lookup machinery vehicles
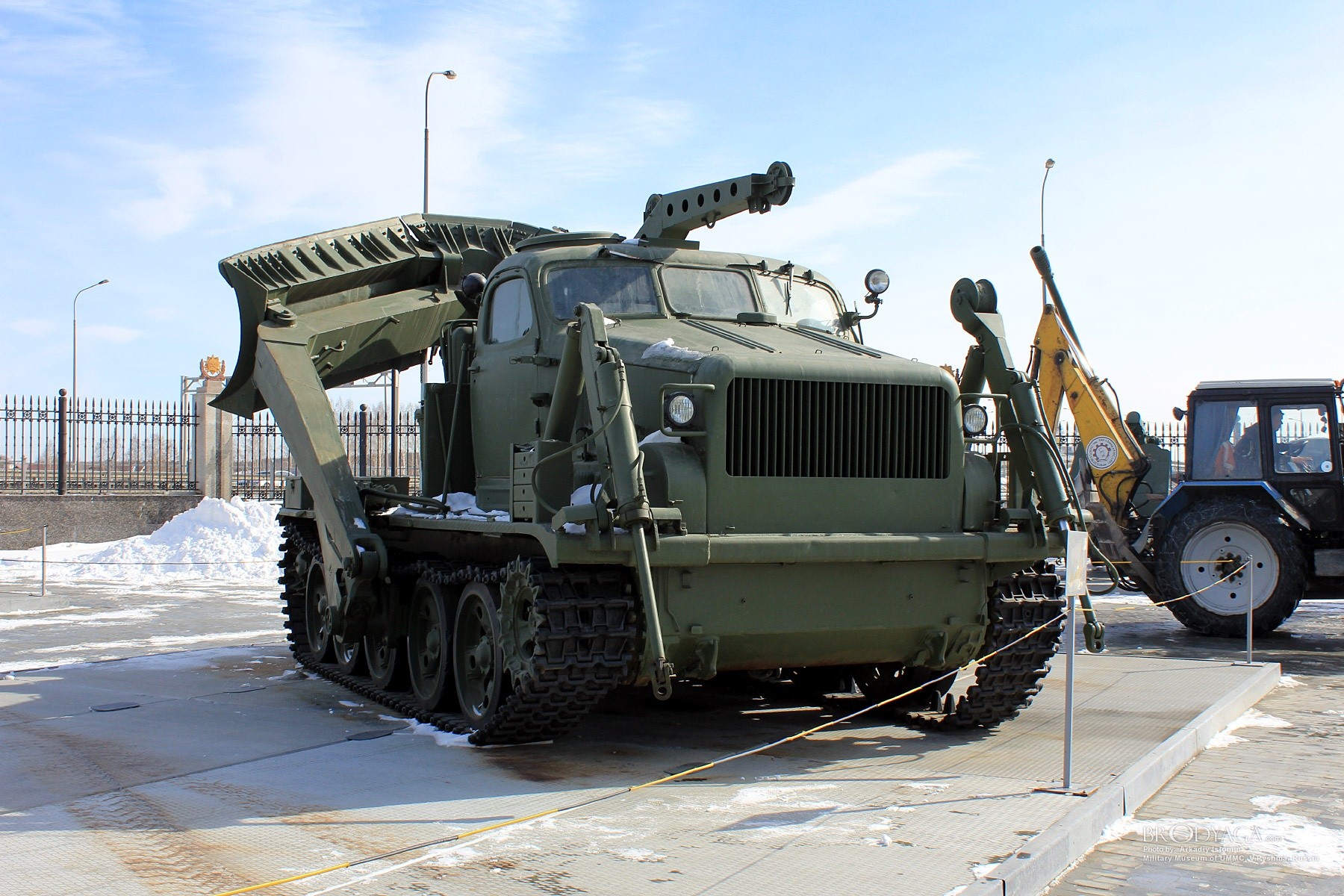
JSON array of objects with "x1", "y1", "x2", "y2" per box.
[
  {"x1": 206, "y1": 159, "x2": 1105, "y2": 749},
  {"x1": 1016, "y1": 301, "x2": 1344, "y2": 639}
]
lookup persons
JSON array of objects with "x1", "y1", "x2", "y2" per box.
[{"x1": 1234, "y1": 408, "x2": 1313, "y2": 478}]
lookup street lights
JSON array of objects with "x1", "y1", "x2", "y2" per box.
[
  {"x1": 424, "y1": 70, "x2": 456, "y2": 213},
  {"x1": 1041, "y1": 158, "x2": 1057, "y2": 306},
  {"x1": 72, "y1": 278, "x2": 109, "y2": 471}
]
